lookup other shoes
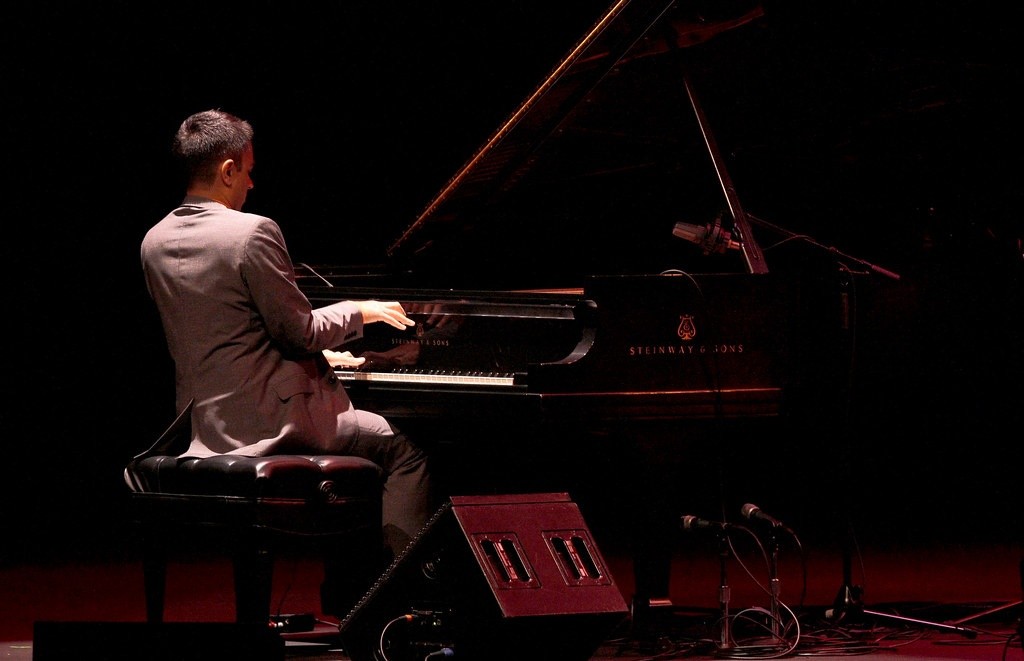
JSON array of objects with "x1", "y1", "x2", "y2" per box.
[
  {"x1": 383, "y1": 558, "x2": 457, "y2": 594},
  {"x1": 320, "y1": 581, "x2": 389, "y2": 615}
]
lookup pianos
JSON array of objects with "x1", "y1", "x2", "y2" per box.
[{"x1": 292, "y1": 1, "x2": 874, "y2": 609}]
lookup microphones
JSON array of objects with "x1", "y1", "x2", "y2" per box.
[
  {"x1": 680, "y1": 515, "x2": 745, "y2": 531},
  {"x1": 673, "y1": 223, "x2": 744, "y2": 251},
  {"x1": 741, "y1": 503, "x2": 793, "y2": 536}
]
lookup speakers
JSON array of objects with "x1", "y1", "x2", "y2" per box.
[{"x1": 337, "y1": 493, "x2": 631, "y2": 661}]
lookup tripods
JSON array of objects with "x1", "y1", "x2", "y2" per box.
[{"x1": 735, "y1": 213, "x2": 977, "y2": 640}]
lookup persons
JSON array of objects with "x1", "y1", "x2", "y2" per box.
[{"x1": 139, "y1": 108, "x2": 454, "y2": 618}]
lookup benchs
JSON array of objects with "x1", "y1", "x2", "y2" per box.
[{"x1": 124, "y1": 455, "x2": 385, "y2": 623}]
